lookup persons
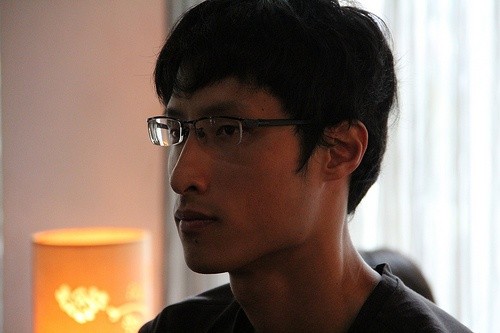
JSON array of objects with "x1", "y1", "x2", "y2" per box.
[{"x1": 137, "y1": 0, "x2": 474, "y2": 333}]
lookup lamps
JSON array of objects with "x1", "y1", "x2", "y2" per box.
[{"x1": 31, "y1": 227, "x2": 155, "y2": 333}]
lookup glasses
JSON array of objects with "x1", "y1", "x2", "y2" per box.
[{"x1": 146, "y1": 113, "x2": 353, "y2": 147}]
